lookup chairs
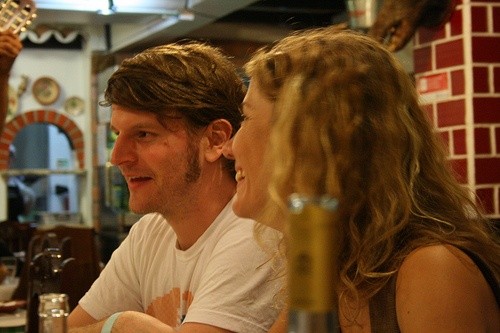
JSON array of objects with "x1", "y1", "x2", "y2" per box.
[
  {"x1": 26, "y1": 225, "x2": 100, "y2": 333},
  {"x1": 0, "y1": 219, "x2": 37, "y2": 257}
]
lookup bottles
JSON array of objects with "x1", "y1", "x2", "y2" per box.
[{"x1": 37, "y1": 293, "x2": 68, "y2": 333}]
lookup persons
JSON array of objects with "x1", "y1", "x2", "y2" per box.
[
  {"x1": 65, "y1": 37, "x2": 284, "y2": 333},
  {"x1": 222, "y1": 24, "x2": 500, "y2": 333}
]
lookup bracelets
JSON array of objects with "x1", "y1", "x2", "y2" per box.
[{"x1": 101, "y1": 312, "x2": 122, "y2": 333}]
